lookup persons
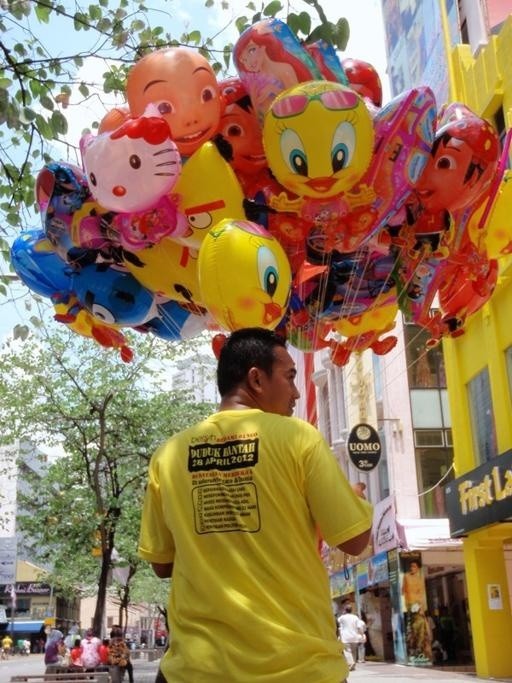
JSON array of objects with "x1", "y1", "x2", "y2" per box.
[
  {"x1": 354, "y1": 611, "x2": 366, "y2": 663},
  {"x1": 137, "y1": 326, "x2": 376, "y2": 681},
  {"x1": 361, "y1": 609, "x2": 376, "y2": 661},
  {"x1": 0, "y1": 622, "x2": 168, "y2": 682},
  {"x1": 400, "y1": 557, "x2": 434, "y2": 654},
  {"x1": 336, "y1": 603, "x2": 366, "y2": 670}
]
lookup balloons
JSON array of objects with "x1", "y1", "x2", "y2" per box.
[{"x1": 11, "y1": 18, "x2": 503, "y2": 365}]
